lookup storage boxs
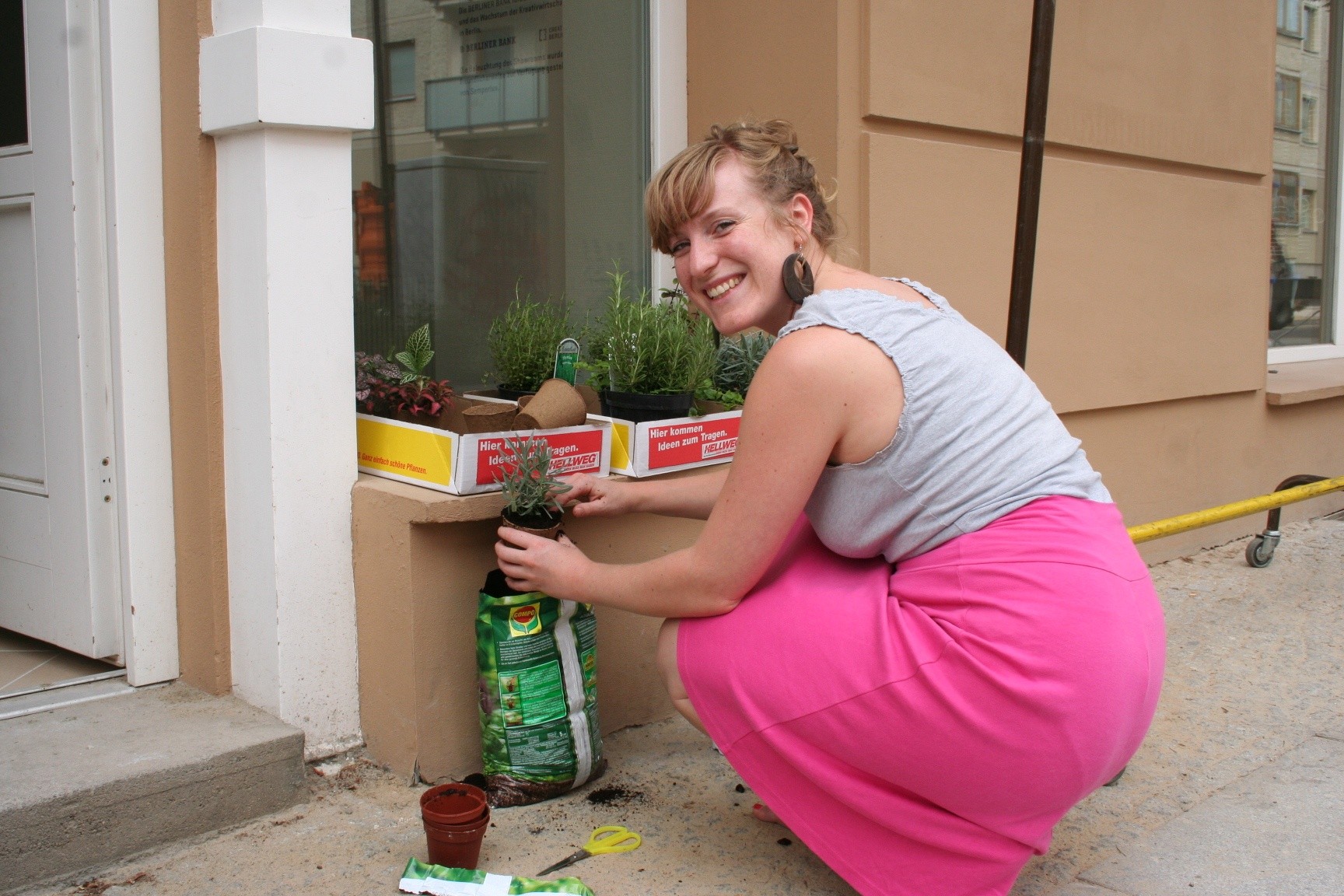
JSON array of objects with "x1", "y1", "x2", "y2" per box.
[
  {"x1": 352, "y1": 391, "x2": 613, "y2": 497},
  {"x1": 462, "y1": 387, "x2": 744, "y2": 477}
]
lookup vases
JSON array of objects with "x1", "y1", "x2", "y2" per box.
[
  {"x1": 419, "y1": 782, "x2": 490, "y2": 870},
  {"x1": 462, "y1": 378, "x2": 588, "y2": 434}
]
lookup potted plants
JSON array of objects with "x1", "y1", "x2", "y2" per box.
[
  {"x1": 492, "y1": 425, "x2": 574, "y2": 562},
  {"x1": 573, "y1": 255, "x2": 718, "y2": 423},
  {"x1": 486, "y1": 275, "x2": 591, "y2": 400},
  {"x1": 378, "y1": 375, "x2": 455, "y2": 428}
]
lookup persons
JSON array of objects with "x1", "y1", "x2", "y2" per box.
[{"x1": 494, "y1": 119, "x2": 1165, "y2": 896}]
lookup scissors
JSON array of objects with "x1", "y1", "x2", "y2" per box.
[{"x1": 536, "y1": 825, "x2": 640, "y2": 877}]
